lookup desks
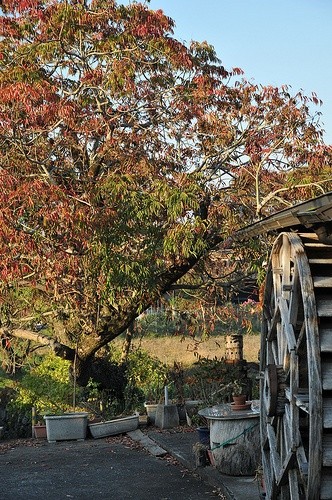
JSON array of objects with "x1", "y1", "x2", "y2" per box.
[{"x1": 198, "y1": 399, "x2": 267, "y2": 476}]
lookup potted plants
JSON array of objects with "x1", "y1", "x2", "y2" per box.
[
  {"x1": 18, "y1": 372, "x2": 180, "y2": 444},
  {"x1": 193, "y1": 416, "x2": 213, "y2": 467},
  {"x1": 230, "y1": 380, "x2": 252, "y2": 410}
]
